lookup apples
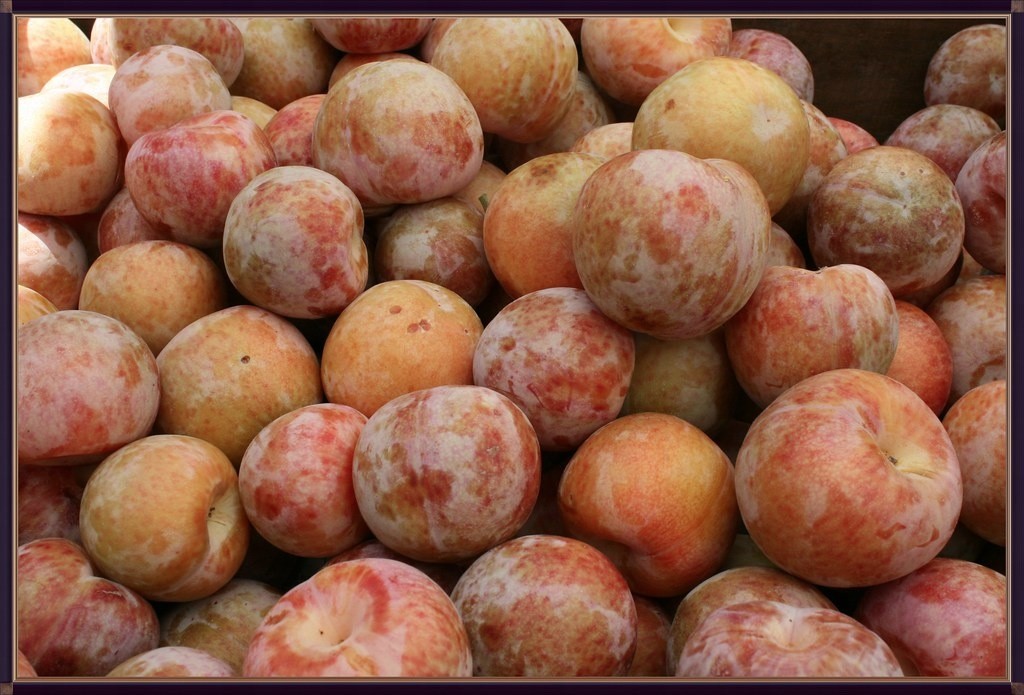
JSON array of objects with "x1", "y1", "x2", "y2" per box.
[{"x1": 16, "y1": 15, "x2": 1010, "y2": 683}]
